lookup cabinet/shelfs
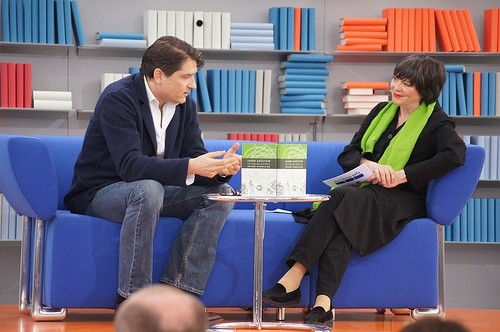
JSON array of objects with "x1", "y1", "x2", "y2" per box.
[
  {"x1": 76, "y1": 45, "x2": 326, "y2": 141},
  {"x1": 0, "y1": 42, "x2": 75, "y2": 243},
  {"x1": 330, "y1": 50, "x2": 500, "y2": 245}
]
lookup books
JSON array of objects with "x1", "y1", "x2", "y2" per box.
[
  {"x1": 1, "y1": 0, "x2": 500, "y2": 143},
  {"x1": 445, "y1": 136, "x2": 500, "y2": 242},
  {"x1": 242, "y1": 143, "x2": 307, "y2": 199}
]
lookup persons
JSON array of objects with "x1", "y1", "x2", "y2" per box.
[
  {"x1": 263, "y1": 53, "x2": 466, "y2": 325},
  {"x1": 63, "y1": 35, "x2": 242, "y2": 310},
  {"x1": 113, "y1": 283, "x2": 208, "y2": 332}
]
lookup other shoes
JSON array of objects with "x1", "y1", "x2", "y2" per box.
[
  {"x1": 262, "y1": 283, "x2": 301, "y2": 307},
  {"x1": 304, "y1": 307, "x2": 333, "y2": 325}
]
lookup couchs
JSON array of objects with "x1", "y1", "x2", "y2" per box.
[{"x1": 0, "y1": 135, "x2": 486, "y2": 320}]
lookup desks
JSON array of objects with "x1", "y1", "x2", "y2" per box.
[{"x1": 200, "y1": 191, "x2": 333, "y2": 332}]
